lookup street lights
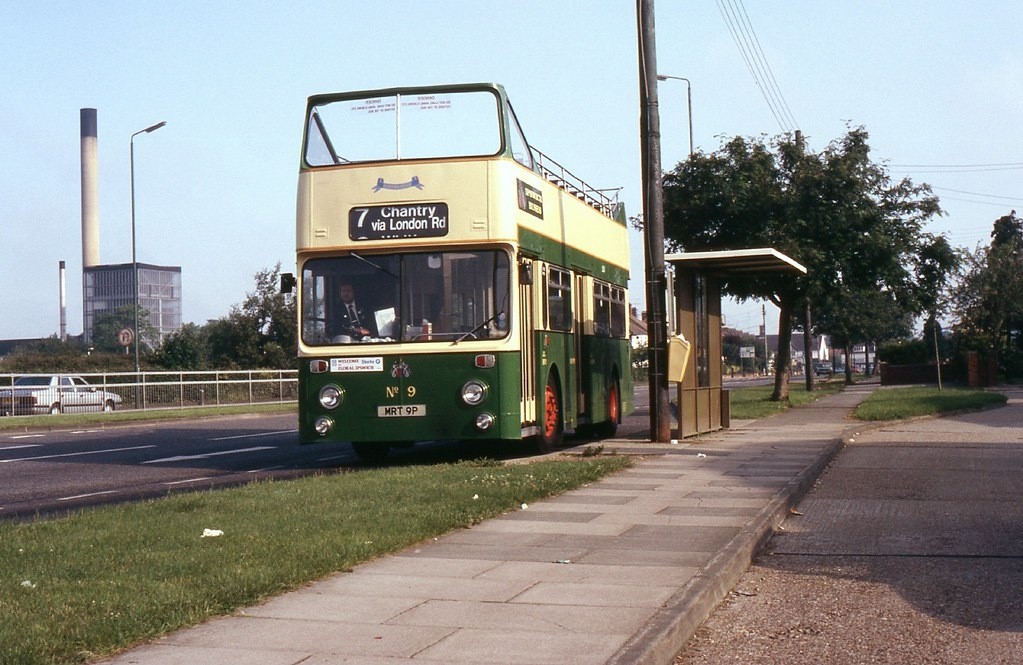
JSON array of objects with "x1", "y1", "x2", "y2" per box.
[
  {"x1": 130, "y1": 121, "x2": 168, "y2": 411},
  {"x1": 658, "y1": 71, "x2": 695, "y2": 171}
]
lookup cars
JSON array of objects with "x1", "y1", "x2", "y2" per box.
[
  {"x1": 817, "y1": 362, "x2": 831, "y2": 376},
  {"x1": 851, "y1": 365, "x2": 863, "y2": 372},
  {"x1": 835, "y1": 368, "x2": 845, "y2": 375},
  {"x1": 0, "y1": 376, "x2": 122, "y2": 416}
]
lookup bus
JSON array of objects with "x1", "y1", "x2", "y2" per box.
[{"x1": 278, "y1": 81, "x2": 635, "y2": 451}]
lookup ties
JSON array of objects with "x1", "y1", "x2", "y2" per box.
[{"x1": 348, "y1": 305, "x2": 361, "y2": 329}]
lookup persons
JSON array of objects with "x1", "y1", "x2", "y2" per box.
[{"x1": 331, "y1": 282, "x2": 378, "y2": 342}]
